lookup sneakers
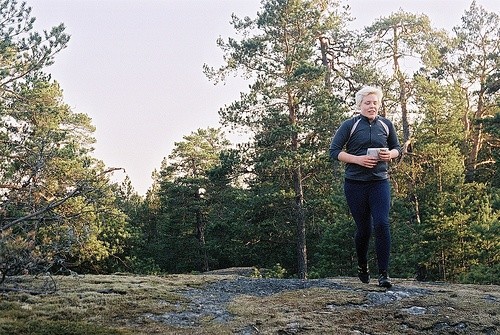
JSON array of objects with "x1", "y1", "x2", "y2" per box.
[
  {"x1": 378, "y1": 270, "x2": 393, "y2": 287},
  {"x1": 357, "y1": 258, "x2": 370, "y2": 284}
]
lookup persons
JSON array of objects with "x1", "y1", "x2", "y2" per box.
[{"x1": 330, "y1": 85, "x2": 401, "y2": 287}]
